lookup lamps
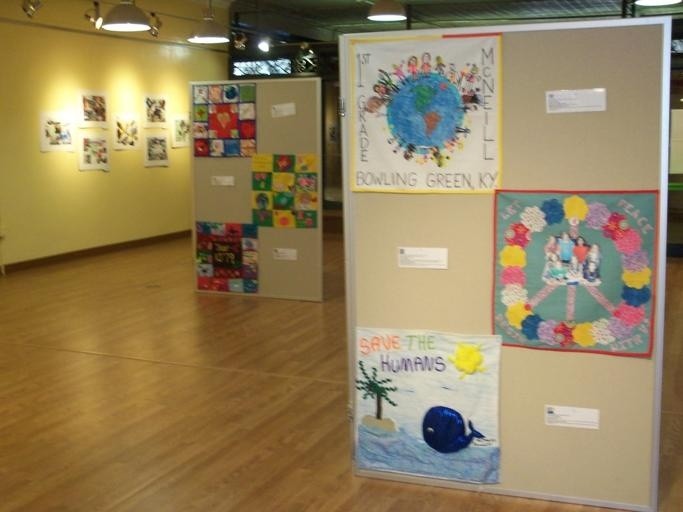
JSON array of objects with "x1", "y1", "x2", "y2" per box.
[
  {"x1": 21, "y1": 1, "x2": 273, "y2": 53},
  {"x1": 365, "y1": 1, "x2": 408, "y2": 22}
]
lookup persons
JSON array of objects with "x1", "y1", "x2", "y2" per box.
[
  {"x1": 537, "y1": 228, "x2": 601, "y2": 289},
  {"x1": 364, "y1": 49, "x2": 481, "y2": 169}
]
